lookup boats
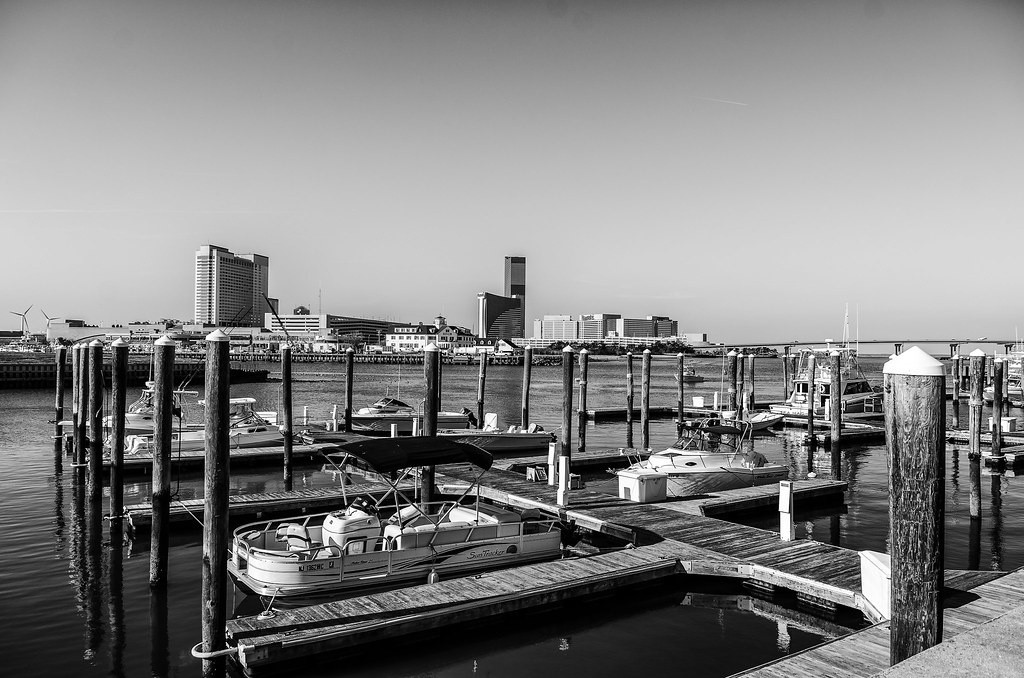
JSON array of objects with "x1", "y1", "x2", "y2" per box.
[
  {"x1": 981, "y1": 326, "x2": 1024, "y2": 405},
  {"x1": 227, "y1": 437, "x2": 567, "y2": 596},
  {"x1": 605, "y1": 414, "x2": 790, "y2": 497},
  {"x1": 53, "y1": 305, "x2": 340, "y2": 469},
  {"x1": 675, "y1": 368, "x2": 709, "y2": 382},
  {"x1": 768, "y1": 305, "x2": 884, "y2": 429},
  {"x1": 676, "y1": 346, "x2": 785, "y2": 439},
  {"x1": 338, "y1": 364, "x2": 557, "y2": 451}
]
